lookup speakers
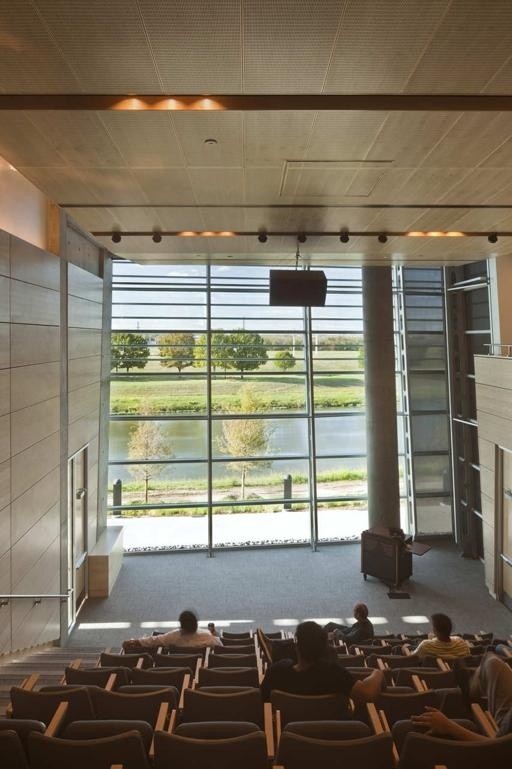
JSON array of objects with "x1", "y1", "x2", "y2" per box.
[{"x1": 270, "y1": 269, "x2": 326, "y2": 306}]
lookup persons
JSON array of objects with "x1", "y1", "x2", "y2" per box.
[
  {"x1": 260, "y1": 622, "x2": 384, "y2": 719},
  {"x1": 322, "y1": 603, "x2": 374, "y2": 646},
  {"x1": 495, "y1": 643, "x2": 512, "y2": 655},
  {"x1": 121, "y1": 612, "x2": 224, "y2": 650},
  {"x1": 411, "y1": 656, "x2": 511, "y2": 743},
  {"x1": 400, "y1": 612, "x2": 472, "y2": 662}
]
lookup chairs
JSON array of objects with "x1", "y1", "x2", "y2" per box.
[{"x1": 1, "y1": 626, "x2": 512, "y2": 768}]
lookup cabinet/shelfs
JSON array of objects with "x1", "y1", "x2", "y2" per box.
[
  {"x1": 88, "y1": 525, "x2": 124, "y2": 598},
  {"x1": 361, "y1": 525, "x2": 432, "y2": 591}
]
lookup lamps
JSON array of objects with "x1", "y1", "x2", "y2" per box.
[{"x1": 90, "y1": 228, "x2": 512, "y2": 244}]
